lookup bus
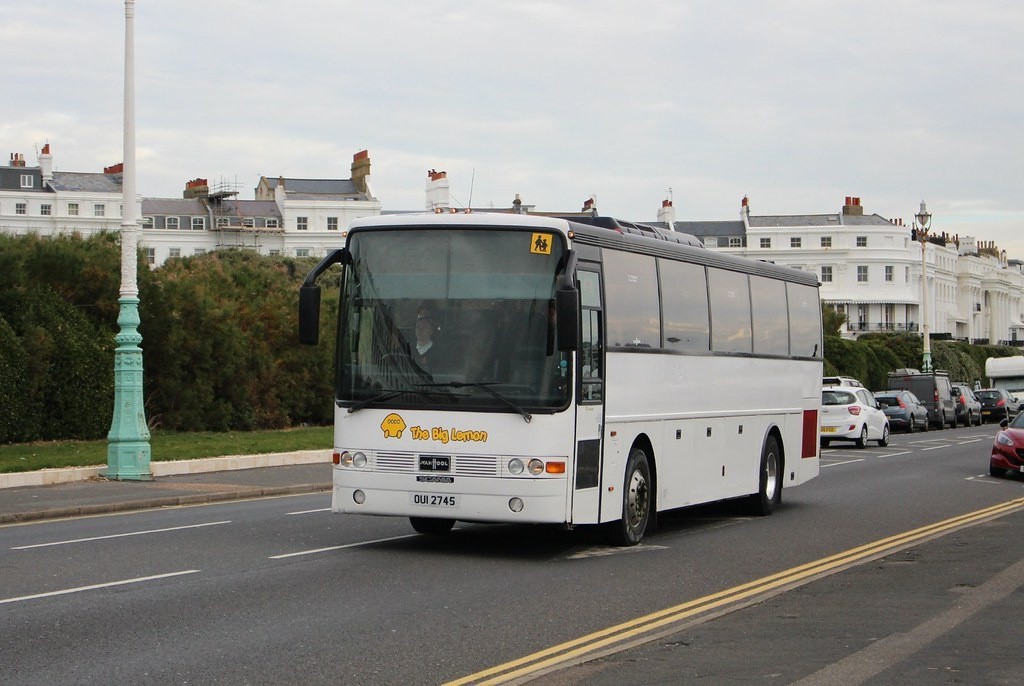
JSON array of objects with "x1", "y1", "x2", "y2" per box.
[{"x1": 300, "y1": 215, "x2": 824, "y2": 543}]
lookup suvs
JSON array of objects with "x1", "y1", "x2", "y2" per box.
[{"x1": 954, "y1": 383, "x2": 981, "y2": 426}]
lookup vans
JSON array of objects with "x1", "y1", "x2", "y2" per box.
[{"x1": 890, "y1": 367, "x2": 956, "y2": 429}]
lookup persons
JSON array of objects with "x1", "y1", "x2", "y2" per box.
[
  {"x1": 414, "y1": 306, "x2": 429, "y2": 323},
  {"x1": 400, "y1": 319, "x2": 440, "y2": 373}
]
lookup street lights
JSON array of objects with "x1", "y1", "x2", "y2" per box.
[{"x1": 913, "y1": 200, "x2": 934, "y2": 374}]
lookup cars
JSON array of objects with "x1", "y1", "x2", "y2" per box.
[
  {"x1": 874, "y1": 388, "x2": 929, "y2": 434},
  {"x1": 822, "y1": 385, "x2": 890, "y2": 450},
  {"x1": 974, "y1": 388, "x2": 1017, "y2": 422},
  {"x1": 989, "y1": 409, "x2": 1023, "y2": 480}
]
